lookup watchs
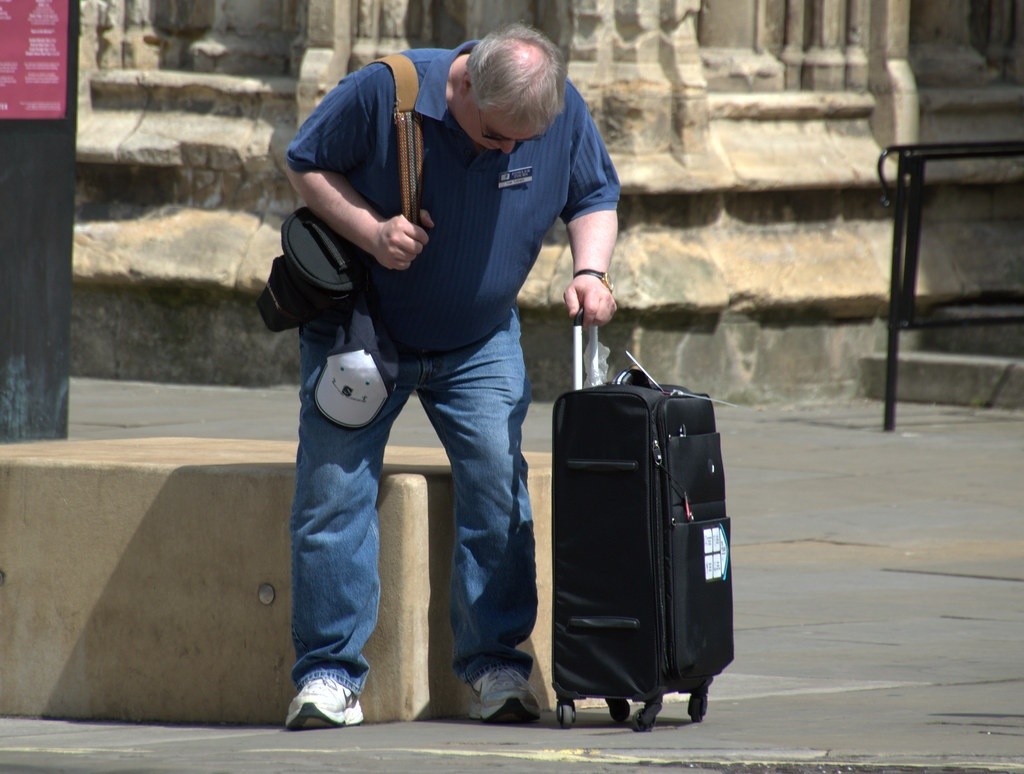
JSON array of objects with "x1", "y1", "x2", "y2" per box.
[{"x1": 574, "y1": 269, "x2": 614, "y2": 294}]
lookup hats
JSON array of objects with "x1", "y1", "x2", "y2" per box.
[{"x1": 311, "y1": 310, "x2": 401, "y2": 429}]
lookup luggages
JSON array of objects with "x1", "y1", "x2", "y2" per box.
[{"x1": 551, "y1": 305, "x2": 734, "y2": 733}]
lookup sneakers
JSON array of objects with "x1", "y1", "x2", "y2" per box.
[
  {"x1": 284, "y1": 675, "x2": 363, "y2": 731},
  {"x1": 468, "y1": 668, "x2": 541, "y2": 724}
]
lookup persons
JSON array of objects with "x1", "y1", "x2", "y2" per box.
[{"x1": 284, "y1": 27, "x2": 619, "y2": 729}]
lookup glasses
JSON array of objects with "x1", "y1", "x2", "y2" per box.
[{"x1": 478, "y1": 108, "x2": 547, "y2": 142}]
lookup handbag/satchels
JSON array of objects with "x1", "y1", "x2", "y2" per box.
[{"x1": 257, "y1": 207, "x2": 370, "y2": 333}]
